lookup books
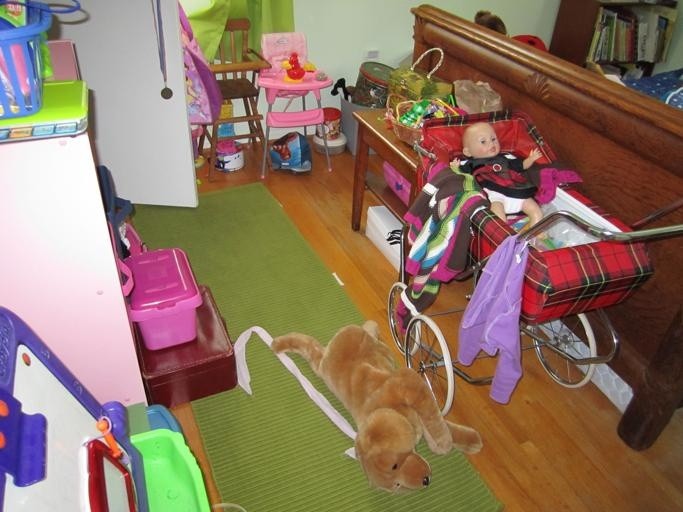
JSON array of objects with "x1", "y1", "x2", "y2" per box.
[{"x1": 588, "y1": 2, "x2": 678, "y2": 86}]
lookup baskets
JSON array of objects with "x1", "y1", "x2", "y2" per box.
[
  {"x1": 0, "y1": 0, "x2": 81, "y2": 120},
  {"x1": 386, "y1": 93, "x2": 450, "y2": 148}
]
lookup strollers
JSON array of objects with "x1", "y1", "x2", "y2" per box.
[{"x1": 388, "y1": 108, "x2": 654, "y2": 416}]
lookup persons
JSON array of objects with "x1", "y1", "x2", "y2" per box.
[{"x1": 450, "y1": 122, "x2": 544, "y2": 232}]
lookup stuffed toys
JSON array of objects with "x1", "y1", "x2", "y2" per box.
[{"x1": 270, "y1": 320, "x2": 482, "y2": 493}]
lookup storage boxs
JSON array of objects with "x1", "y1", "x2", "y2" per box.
[{"x1": 124, "y1": 246, "x2": 237, "y2": 407}]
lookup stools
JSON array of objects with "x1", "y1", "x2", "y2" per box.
[{"x1": 350, "y1": 109, "x2": 421, "y2": 281}]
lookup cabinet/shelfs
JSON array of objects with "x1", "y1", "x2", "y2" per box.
[{"x1": 549, "y1": 2, "x2": 669, "y2": 81}]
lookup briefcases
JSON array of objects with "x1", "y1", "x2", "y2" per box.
[{"x1": 132, "y1": 284, "x2": 238, "y2": 407}]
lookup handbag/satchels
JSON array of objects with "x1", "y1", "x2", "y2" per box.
[
  {"x1": 177, "y1": 0, "x2": 223, "y2": 125},
  {"x1": 388, "y1": 47, "x2": 453, "y2": 117},
  {"x1": 269, "y1": 131, "x2": 312, "y2": 175}
]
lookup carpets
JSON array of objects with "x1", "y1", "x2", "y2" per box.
[{"x1": 130, "y1": 181, "x2": 505, "y2": 511}]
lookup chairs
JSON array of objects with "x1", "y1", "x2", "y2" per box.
[{"x1": 196, "y1": 16, "x2": 337, "y2": 183}]
